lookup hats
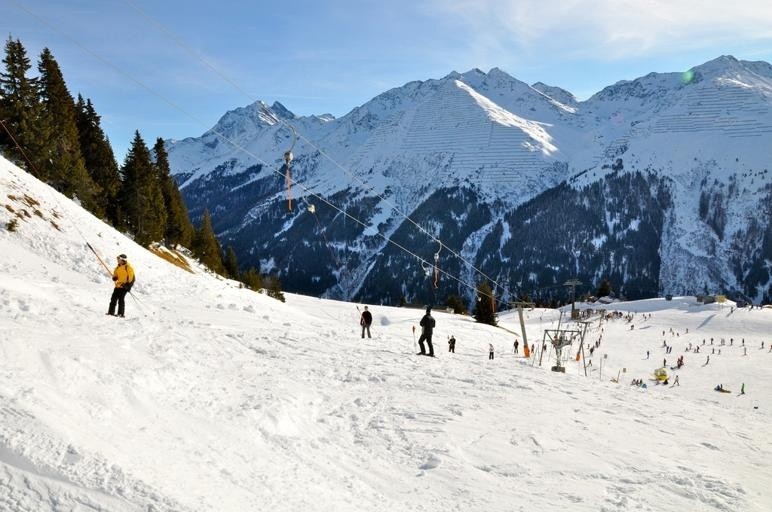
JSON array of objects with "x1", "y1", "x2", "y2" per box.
[{"x1": 118, "y1": 254, "x2": 127, "y2": 261}]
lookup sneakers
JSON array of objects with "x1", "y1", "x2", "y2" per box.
[
  {"x1": 115, "y1": 313, "x2": 125, "y2": 317},
  {"x1": 417, "y1": 351, "x2": 434, "y2": 356}
]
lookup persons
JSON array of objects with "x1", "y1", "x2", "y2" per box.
[
  {"x1": 448, "y1": 336, "x2": 455, "y2": 352},
  {"x1": 513, "y1": 339, "x2": 519, "y2": 353},
  {"x1": 585, "y1": 304, "x2": 772, "y2": 397},
  {"x1": 489, "y1": 343, "x2": 494, "y2": 359},
  {"x1": 416, "y1": 307, "x2": 435, "y2": 356},
  {"x1": 105, "y1": 254, "x2": 137, "y2": 317},
  {"x1": 360, "y1": 306, "x2": 373, "y2": 338}
]
